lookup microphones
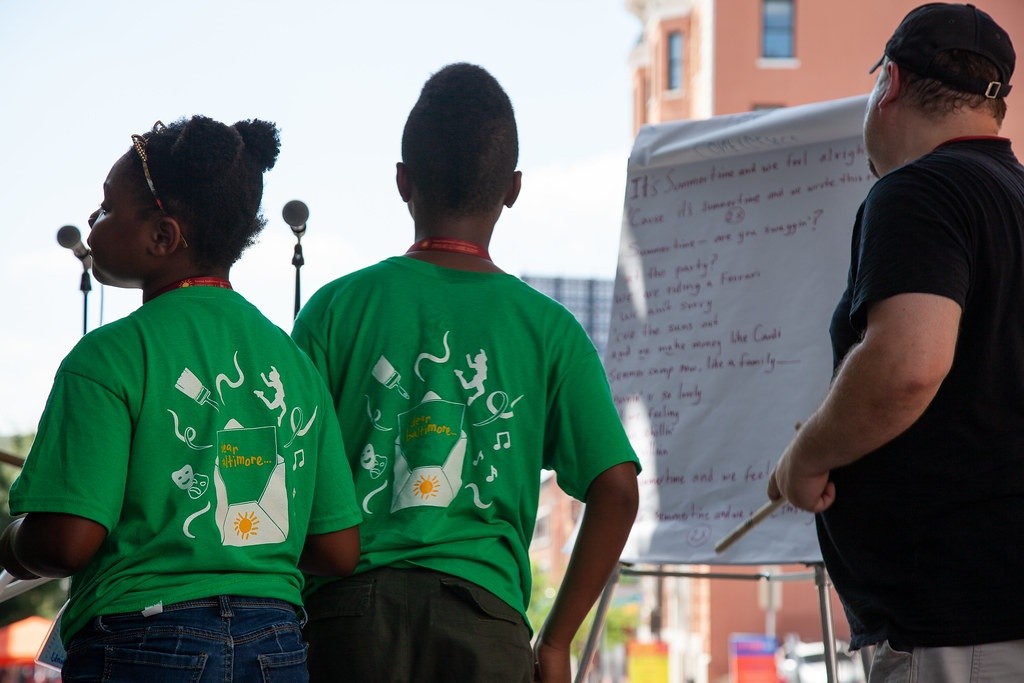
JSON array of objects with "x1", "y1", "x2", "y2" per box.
[
  {"x1": 282, "y1": 200, "x2": 309, "y2": 237},
  {"x1": 57, "y1": 225, "x2": 92, "y2": 269}
]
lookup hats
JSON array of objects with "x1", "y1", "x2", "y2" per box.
[{"x1": 870, "y1": 2, "x2": 1017, "y2": 98}]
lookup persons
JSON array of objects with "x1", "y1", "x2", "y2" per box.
[
  {"x1": 0, "y1": 114, "x2": 362, "y2": 683},
  {"x1": 768, "y1": 3, "x2": 1024, "y2": 683},
  {"x1": 292, "y1": 63, "x2": 644, "y2": 683}
]
